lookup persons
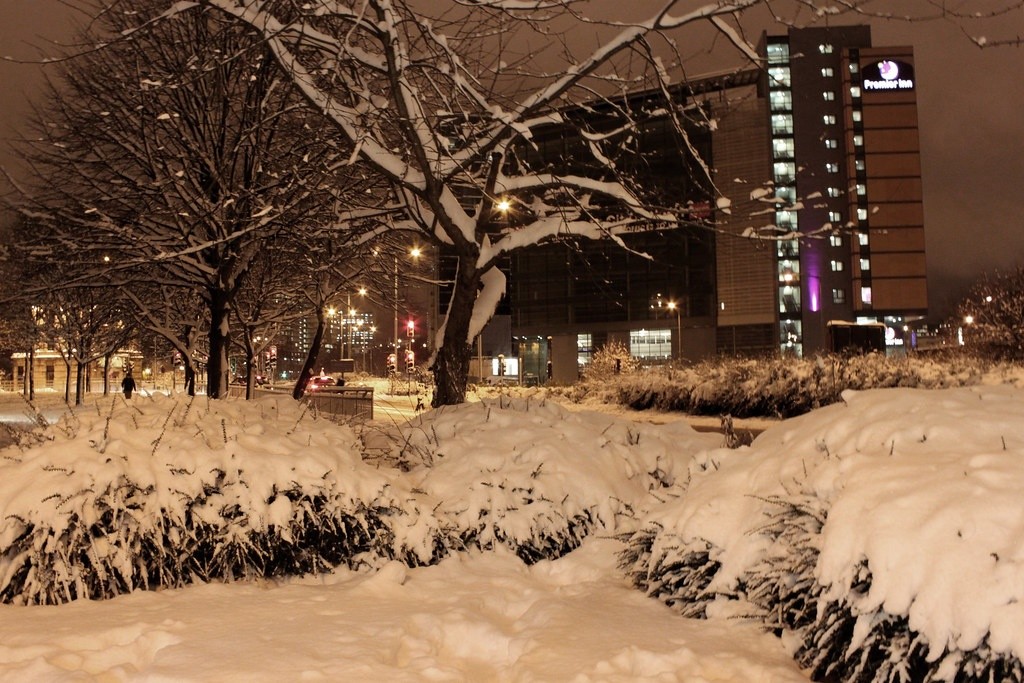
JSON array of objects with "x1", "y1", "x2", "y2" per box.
[{"x1": 121, "y1": 373, "x2": 136, "y2": 399}]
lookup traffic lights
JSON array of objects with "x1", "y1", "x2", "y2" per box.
[
  {"x1": 406, "y1": 321, "x2": 415, "y2": 338},
  {"x1": 388, "y1": 353, "x2": 397, "y2": 375},
  {"x1": 405, "y1": 350, "x2": 415, "y2": 374}
]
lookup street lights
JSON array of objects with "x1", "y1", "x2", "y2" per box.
[
  {"x1": 667, "y1": 301, "x2": 682, "y2": 359},
  {"x1": 328, "y1": 309, "x2": 344, "y2": 359},
  {"x1": 347, "y1": 286, "x2": 366, "y2": 358}
]
